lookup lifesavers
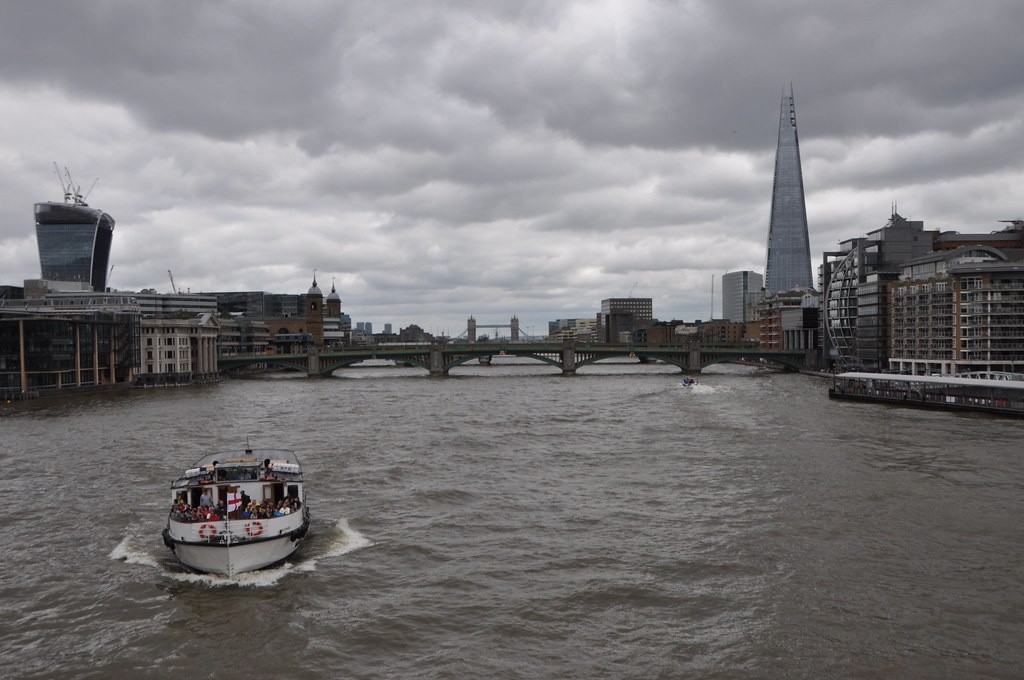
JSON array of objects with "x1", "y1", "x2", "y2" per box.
[
  {"x1": 246, "y1": 521, "x2": 263, "y2": 536},
  {"x1": 199, "y1": 524, "x2": 216, "y2": 539}
]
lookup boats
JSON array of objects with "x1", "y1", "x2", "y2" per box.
[
  {"x1": 162, "y1": 448, "x2": 310, "y2": 578},
  {"x1": 682, "y1": 376, "x2": 695, "y2": 387}
]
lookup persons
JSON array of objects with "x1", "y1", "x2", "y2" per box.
[
  {"x1": 237, "y1": 468, "x2": 251, "y2": 480},
  {"x1": 274, "y1": 496, "x2": 300, "y2": 517},
  {"x1": 170, "y1": 494, "x2": 192, "y2": 523},
  {"x1": 200, "y1": 488, "x2": 213, "y2": 507},
  {"x1": 192, "y1": 501, "x2": 227, "y2": 523},
  {"x1": 228, "y1": 490, "x2": 274, "y2": 521}
]
